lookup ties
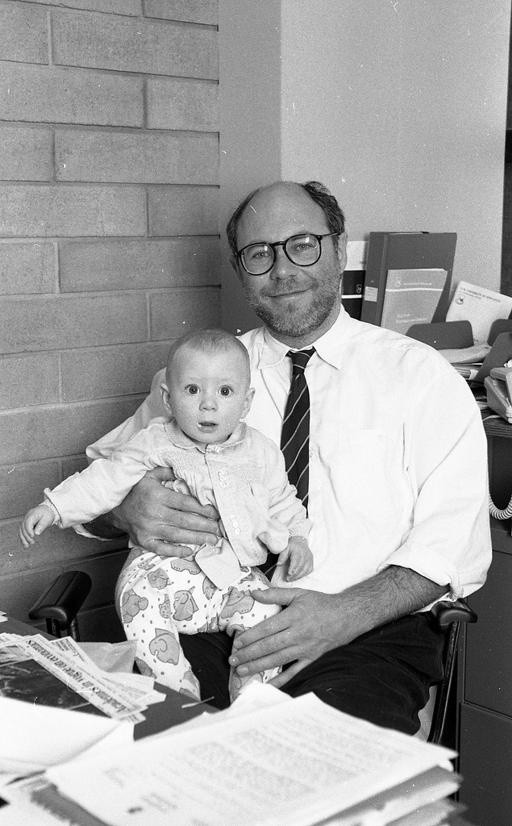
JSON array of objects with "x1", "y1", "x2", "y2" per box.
[{"x1": 254, "y1": 346, "x2": 321, "y2": 586}]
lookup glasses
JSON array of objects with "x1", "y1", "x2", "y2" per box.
[{"x1": 232, "y1": 228, "x2": 340, "y2": 279}]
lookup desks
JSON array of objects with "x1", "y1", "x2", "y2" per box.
[
  {"x1": 474, "y1": 406, "x2": 512, "y2": 518},
  {"x1": 0, "y1": 613, "x2": 473, "y2": 826}
]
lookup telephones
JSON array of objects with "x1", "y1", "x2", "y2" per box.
[{"x1": 484, "y1": 367, "x2": 512, "y2": 423}]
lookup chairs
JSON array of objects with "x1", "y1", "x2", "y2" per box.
[{"x1": 22, "y1": 559, "x2": 476, "y2": 819}]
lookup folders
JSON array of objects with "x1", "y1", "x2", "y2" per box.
[{"x1": 360, "y1": 231, "x2": 457, "y2": 335}]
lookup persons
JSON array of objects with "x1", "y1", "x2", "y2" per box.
[
  {"x1": 86, "y1": 178, "x2": 493, "y2": 736},
  {"x1": 18, "y1": 327, "x2": 315, "y2": 705}
]
